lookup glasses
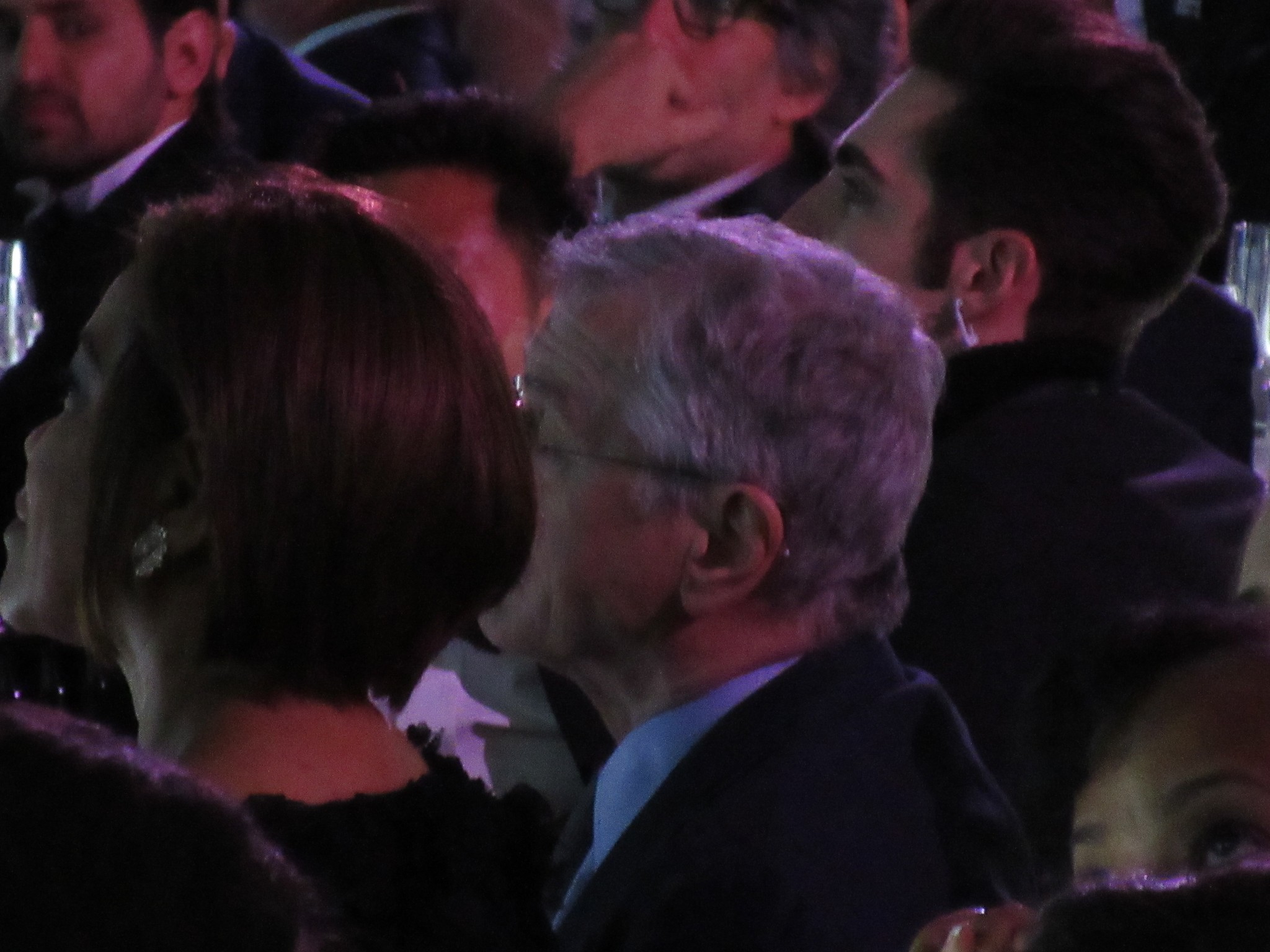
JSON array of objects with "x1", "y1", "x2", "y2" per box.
[
  {"x1": 598, "y1": 0, "x2": 800, "y2": 36},
  {"x1": 509, "y1": 371, "x2": 718, "y2": 489}
]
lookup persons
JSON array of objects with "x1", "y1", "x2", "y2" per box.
[
  {"x1": 2, "y1": 698, "x2": 350, "y2": 950},
  {"x1": 296, "y1": 86, "x2": 624, "y2": 883},
  {"x1": 1113, "y1": 271, "x2": 1263, "y2": 491},
  {"x1": 0, "y1": 168, "x2": 571, "y2": 951},
  {"x1": 1054, "y1": 591, "x2": 1270, "y2": 896},
  {"x1": 516, "y1": 0, "x2": 881, "y2": 233},
  {"x1": 1, "y1": 0, "x2": 262, "y2": 741},
  {"x1": 899, "y1": 860, "x2": 1270, "y2": 952},
  {"x1": 468, "y1": 204, "x2": 1043, "y2": 951},
  {"x1": 765, "y1": 0, "x2": 1269, "y2": 810}
]
